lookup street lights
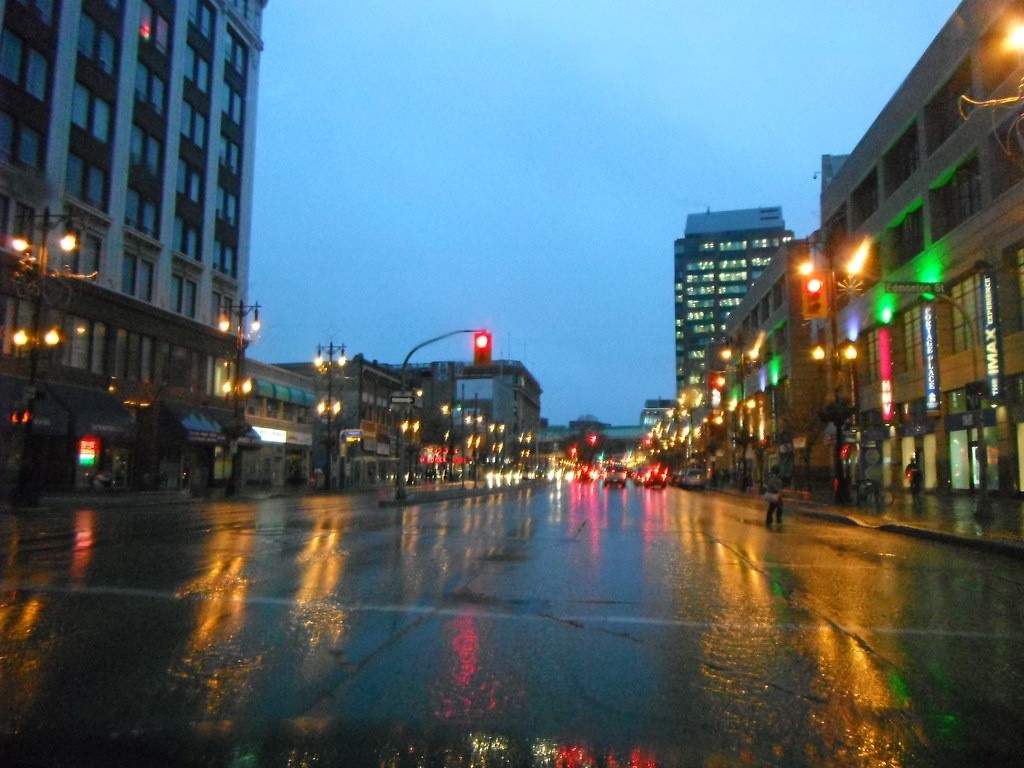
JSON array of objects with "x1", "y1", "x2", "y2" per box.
[
  {"x1": 11, "y1": 205, "x2": 98, "y2": 510},
  {"x1": 317, "y1": 341, "x2": 349, "y2": 494},
  {"x1": 844, "y1": 261, "x2": 989, "y2": 524},
  {"x1": 214, "y1": 299, "x2": 265, "y2": 501}
]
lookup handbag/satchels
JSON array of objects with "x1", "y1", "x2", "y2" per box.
[{"x1": 764, "y1": 493, "x2": 778, "y2": 503}]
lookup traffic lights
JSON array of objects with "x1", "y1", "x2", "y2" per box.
[
  {"x1": 473, "y1": 331, "x2": 492, "y2": 369},
  {"x1": 803, "y1": 268, "x2": 832, "y2": 320}
]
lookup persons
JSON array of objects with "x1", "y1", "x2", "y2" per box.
[
  {"x1": 905, "y1": 457, "x2": 925, "y2": 505},
  {"x1": 764, "y1": 465, "x2": 785, "y2": 531}
]
